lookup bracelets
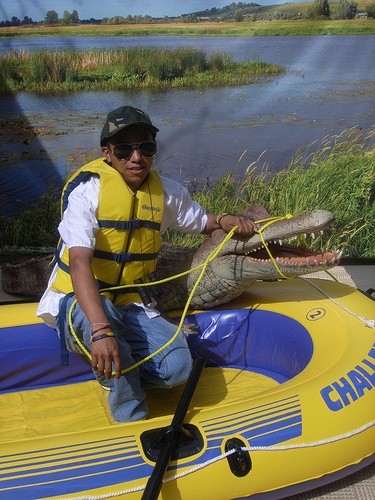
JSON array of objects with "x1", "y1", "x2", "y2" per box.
[
  {"x1": 89, "y1": 324, "x2": 113, "y2": 336},
  {"x1": 89, "y1": 331, "x2": 115, "y2": 346},
  {"x1": 216, "y1": 211, "x2": 234, "y2": 226}
]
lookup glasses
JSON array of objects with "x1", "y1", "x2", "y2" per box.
[{"x1": 107, "y1": 142, "x2": 157, "y2": 161}]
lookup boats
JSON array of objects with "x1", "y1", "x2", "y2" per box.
[{"x1": 1, "y1": 277, "x2": 375, "y2": 500}]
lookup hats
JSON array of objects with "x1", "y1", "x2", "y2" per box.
[{"x1": 99, "y1": 106, "x2": 159, "y2": 146}]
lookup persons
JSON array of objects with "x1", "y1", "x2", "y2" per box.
[{"x1": 34, "y1": 104, "x2": 258, "y2": 422}]
